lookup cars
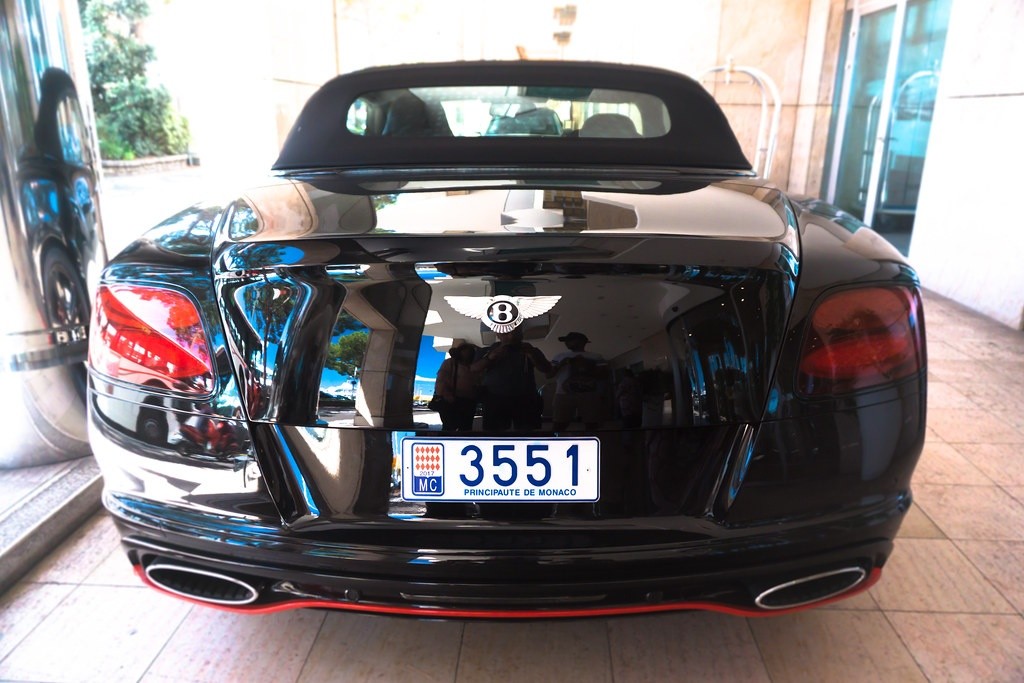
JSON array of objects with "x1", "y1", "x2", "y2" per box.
[{"x1": 87, "y1": 60, "x2": 928, "y2": 615}]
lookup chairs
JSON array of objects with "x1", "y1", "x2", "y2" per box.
[
  {"x1": 579, "y1": 113, "x2": 636, "y2": 137},
  {"x1": 381, "y1": 94, "x2": 454, "y2": 137}
]
